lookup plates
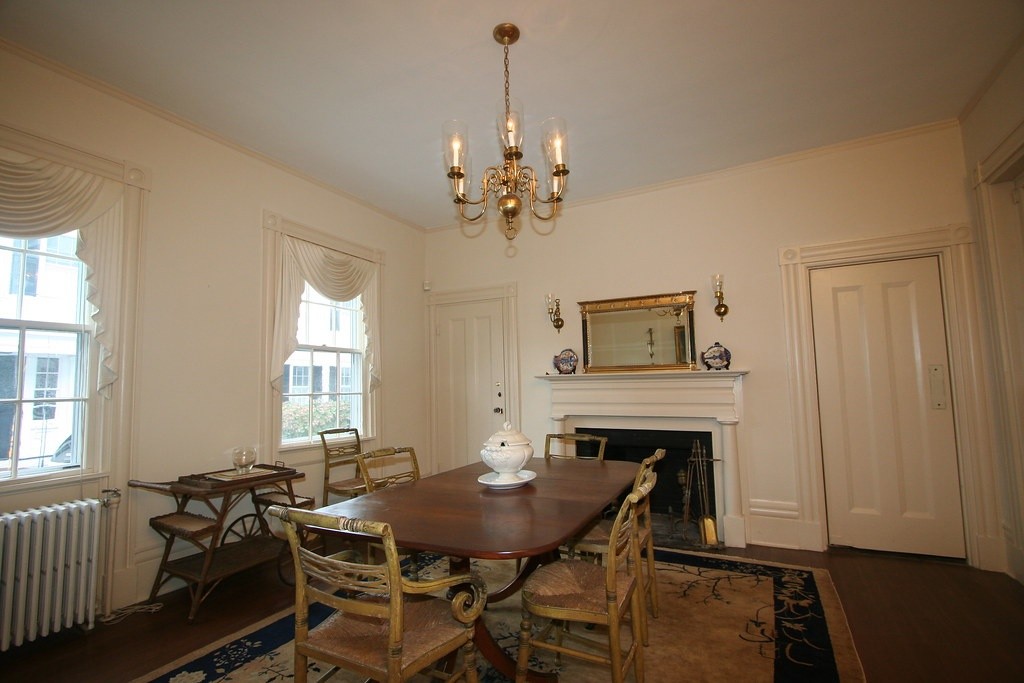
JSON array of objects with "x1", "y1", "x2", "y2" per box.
[{"x1": 478, "y1": 470, "x2": 537, "y2": 489}]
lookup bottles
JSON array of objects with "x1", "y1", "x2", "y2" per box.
[{"x1": 480, "y1": 420, "x2": 534, "y2": 482}]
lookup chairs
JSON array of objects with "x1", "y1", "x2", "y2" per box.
[
  {"x1": 318, "y1": 428, "x2": 388, "y2": 507},
  {"x1": 354, "y1": 447, "x2": 426, "y2": 589},
  {"x1": 514, "y1": 472, "x2": 658, "y2": 683},
  {"x1": 268, "y1": 505, "x2": 488, "y2": 683},
  {"x1": 567, "y1": 448, "x2": 666, "y2": 647},
  {"x1": 544, "y1": 433, "x2": 607, "y2": 561}
]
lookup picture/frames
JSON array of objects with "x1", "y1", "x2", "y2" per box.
[{"x1": 674, "y1": 325, "x2": 686, "y2": 365}]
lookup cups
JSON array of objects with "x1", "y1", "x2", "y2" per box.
[{"x1": 233, "y1": 447, "x2": 256, "y2": 474}]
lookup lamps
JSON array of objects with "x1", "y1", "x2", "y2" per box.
[
  {"x1": 676, "y1": 469, "x2": 687, "y2": 496},
  {"x1": 656, "y1": 307, "x2": 687, "y2": 326},
  {"x1": 712, "y1": 274, "x2": 729, "y2": 323},
  {"x1": 544, "y1": 293, "x2": 564, "y2": 334},
  {"x1": 442, "y1": 24, "x2": 570, "y2": 229}
]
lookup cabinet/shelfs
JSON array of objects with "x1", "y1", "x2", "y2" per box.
[{"x1": 128, "y1": 464, "x2": 315, "y2": 625}]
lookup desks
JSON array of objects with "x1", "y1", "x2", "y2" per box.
[{"x1": 303, "y1": 457, "x2": 641, "y2": 683}]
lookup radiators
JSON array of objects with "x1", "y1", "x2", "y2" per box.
[{"x1": 0, "y1": 498, "x2": 102, "y2": 652}]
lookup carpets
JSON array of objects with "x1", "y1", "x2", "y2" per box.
[{"x1": 127, "y1": 541, "x2": 868, "y2": 683}]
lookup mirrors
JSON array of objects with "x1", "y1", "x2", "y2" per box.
[{"x1": 577, "y1": 291, "x2": 698, "y2": 373}]
